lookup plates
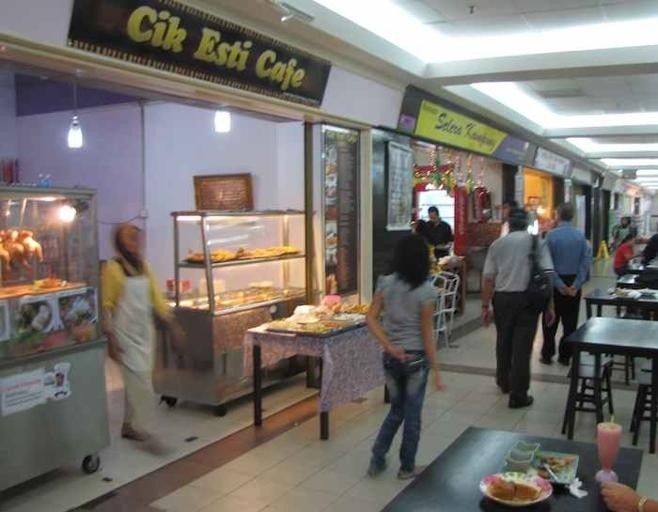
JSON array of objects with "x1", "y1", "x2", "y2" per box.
[
  {"x1": 503, "y1": 439, "x2": 540, "y2": 466},
  {"x1": 613, "y1": 290, "x2": 640, "y2": 299},
  {"x1": 480, "y1": 471, "x2": 554, "y2": 508},
  {"x1": 639, "y1": 289, "x2": 657, "y2": 296},
  {"x1": 497, "y1": 450, "x2": 580, "y2": 485},
  {"x1": 17, "y1": 299, "x2": 55, "y2": 333}
]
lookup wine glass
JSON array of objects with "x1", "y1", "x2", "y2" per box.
[{"x1": 594, "y1": 423, "x2": 624, "y2": 484}]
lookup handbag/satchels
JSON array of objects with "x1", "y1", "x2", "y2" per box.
[{"x1": 526, "y1": 233, "x2": 552, "y2": 314}]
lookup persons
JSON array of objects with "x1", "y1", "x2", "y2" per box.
[
  {"x1": 365, "y1": 234, "x2": 447, "y2": 480},
  {"x1": 611, "y1": 217, "x2": 631, "y2": 249},
  {"x1": 600, "y1": 480, "x2": 658, "y2": 512},
  {"x1": 422, "y1": 205, "x2": 453, "y2": 257},
  {"x1": 501, "y1": 199, "x2": 517, "y2": 236},
  {"x1": 539, "y1": 202, "x2": 591, "y2": 364},
  {"x1": 99, "y1": 223, "x2": 170, "y2": 439},
  {"x1": 646, "y1": 234, "x2": 658, "y2": 264},
  {"x1": 613, "y1": 233, "x2": 639, "y2": 275},
  {"x1": 479, "y1": 210, "x2": 555, "y2": 408}
]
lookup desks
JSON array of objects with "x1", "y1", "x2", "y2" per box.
[{"x1": 239, "y1": 311, "x2": 390, "y2": 442}]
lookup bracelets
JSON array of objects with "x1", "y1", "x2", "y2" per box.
[
  {"x1": 638, "y1": 495, "x2": 648, "y2": 511},
  {"x1": 481, "y1": 305, "x2": 490, "y2": 308}
]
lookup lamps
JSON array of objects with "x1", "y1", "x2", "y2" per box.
[
  {"x1": 58, "y1": 197, "x2": 90, "y2": 221},
  {"x1": 215, "y1": 110, "x2": 231, "y2": 134},
  {"x1": 66, "y1": 82, "x2": 83, "y2": 149}
]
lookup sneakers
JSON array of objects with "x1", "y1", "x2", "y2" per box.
[
  {"x1": 501, "y1": 385, "x2": 534, "y2": 408},
  {"x1": 367, "y1": 462, "x2": 389, "y2": 477},
  {"x1": 121, "y1": 422, "x2": 152, "y2": 442},
  {"x1": 397, "y1": 464, "x2": 428, "y2": 480},
  {"x1": 556, "y1": 356, "x2": 570, "y2": 367},
  {"x1": 539, "y1": 357, "x2": 553, "y2": 365}
]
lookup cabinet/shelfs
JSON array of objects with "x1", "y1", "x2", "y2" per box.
[
  {"x1": 154, "y1": 208, "x2": 305, "y2": 422},
  {"x1": 0, "y1": 182, "x2": 111, "y2": 495}
]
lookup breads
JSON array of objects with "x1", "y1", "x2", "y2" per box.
[{"x1": 489, "y1": 478, "x2": 543, "y2": 501}]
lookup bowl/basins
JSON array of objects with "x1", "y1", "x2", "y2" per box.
[{"x1": 294, "y1": 304, "x2": 319, "y2": 323}]
